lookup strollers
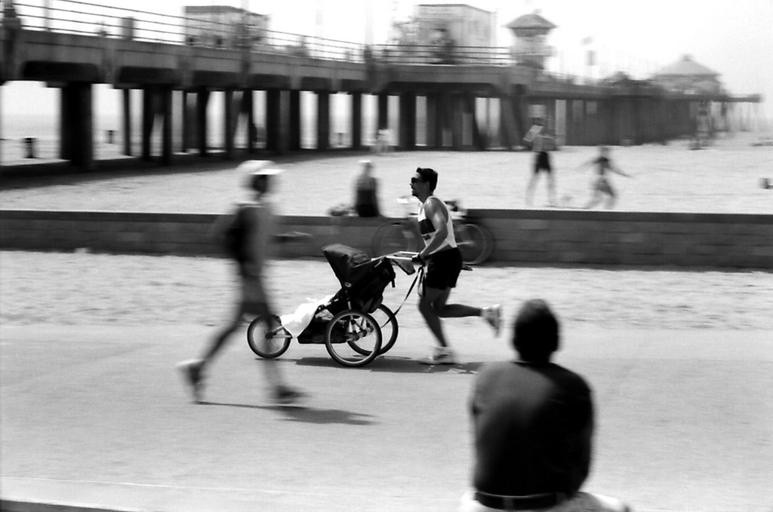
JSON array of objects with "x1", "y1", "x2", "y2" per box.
[{"x1": 239, "y1": 241, "x2": 429, "y2": 372}]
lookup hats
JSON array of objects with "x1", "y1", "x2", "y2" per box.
[
  {"x1": 235, "y1": 158, "x2": 285, "y2": 178},
  {"x1": 414, "y1": 167, "x2": 438, "y2": 190}
]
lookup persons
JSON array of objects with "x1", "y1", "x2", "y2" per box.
[
  {"x1": 522, "y1": 122, "x2": 564, "y2": 208},
  {"x1": 173, "y1": 159, "x2": 315, "y2": 405},
  {"x1": 353, "y1": 161, "x2": 384, "y2": 218},
  {"x1": 411, "y1": 166, "x2": 500, "y2": 365},
  {"x1": 469, "y1": 295, "x2": 596, "y2": 512},
  {"x1": 570, "y1": 144, "x2": 633, "y2": 210}
]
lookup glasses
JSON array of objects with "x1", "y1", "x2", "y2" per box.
[{"x1": 410, "y1": 177, "x2": 423, "y2": 183}]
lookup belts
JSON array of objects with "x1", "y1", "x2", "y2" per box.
[{"x1": 469, "y1": 488, "x2": 560, "y2": 511}]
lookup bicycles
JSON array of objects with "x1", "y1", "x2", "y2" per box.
[{"x1": 368, "y1": 186, "x2": 498, "y2": 266}]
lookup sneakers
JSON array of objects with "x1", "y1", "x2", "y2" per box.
[
  {"x1": 260, "y1": 384, "x2": 305, "y2": 404},
  {"x1": 167, "y1": 359, "x2": 203, "y2": 404},
  {"x1": 485, "y1": 303, "x2": 502, "y2": 337},
  {"x1": 416, "y1": 346, "x2": 456, "y2": 365}
]
